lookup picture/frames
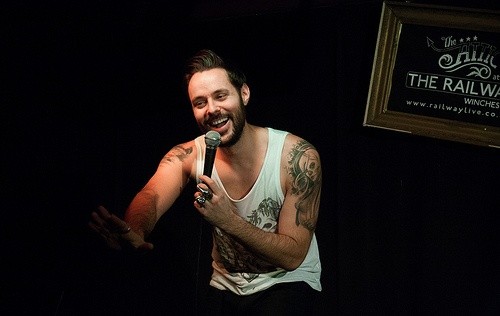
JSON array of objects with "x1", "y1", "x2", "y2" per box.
[{"x1": 363, "y1": 0, "x2": 500, "y2": 149}]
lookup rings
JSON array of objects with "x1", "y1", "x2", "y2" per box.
[
  {"x1": 201, "y1": 190, "x2": 213, "y2": 201},
  {"x1": 196, "y1": 198, "x2": 206, "y2": 206},
  {"x1": 118, "y1": 224, "x2": 131, "y2": 234}
]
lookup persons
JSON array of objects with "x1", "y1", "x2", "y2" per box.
[{"x1": 88, "y1": 48, "x2": 322, "y2": 316}]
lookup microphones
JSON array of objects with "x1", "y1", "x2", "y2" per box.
[{"x1": 202, "y1": 130, "x2": 221, "y2": 188}]
